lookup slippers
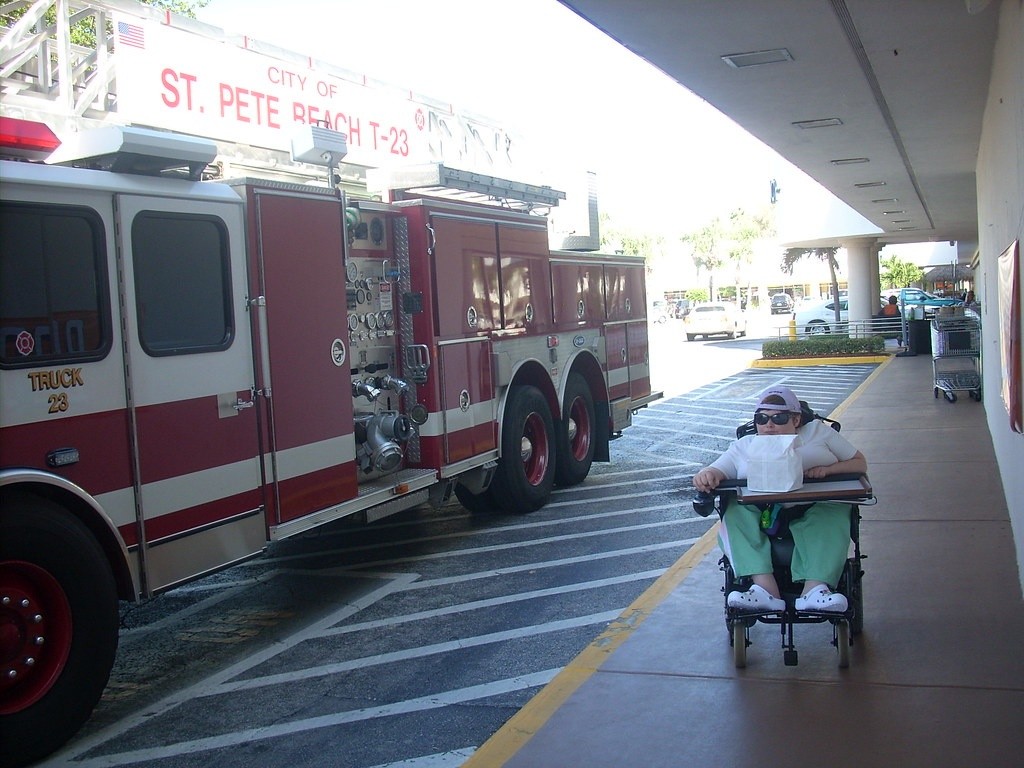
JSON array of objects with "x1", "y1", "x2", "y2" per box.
[
  {"x1": 727, "y1": 584, "x2": 786, "y2": 611},
  {"x1": 795, "y1": 585, "x2": 848, "y2": 612}
]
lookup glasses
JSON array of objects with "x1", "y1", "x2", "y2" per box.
[{"x1": 754, "y1": 413, "x2": 794, "y2": 425}]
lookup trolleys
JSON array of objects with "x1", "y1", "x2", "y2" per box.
[{"x1": 930, "y1": 304, "x2": 981, "y2": 402}]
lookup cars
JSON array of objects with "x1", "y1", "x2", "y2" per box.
[
  {"x1": 770, "y1": 294, "x2": 795, "y2": 315},
  {"x1": 804, "y1": 288, "x2": 965, "y2": 336},
  {"x1": 653, "y1": 299, "x2": 689, "y2": 324},
  {"x1": 685, "y1": 300, "x2": 747, "y2": 341}
]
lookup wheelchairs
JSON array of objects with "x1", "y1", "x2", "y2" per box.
[{"x1": 693, "y1": 400, "x2": 878, "y2": 669}]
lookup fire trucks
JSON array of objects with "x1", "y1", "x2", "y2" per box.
[{"x1": 0, "y1": 116, "x2": 664, "y2": 768}]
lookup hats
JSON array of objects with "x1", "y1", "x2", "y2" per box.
[{"x1": 755, "y1": 386, "x2": 802, "y2": 414}]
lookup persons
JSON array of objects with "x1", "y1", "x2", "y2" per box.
[
  {"x1": 691, "y1": 385, "x2": 867, "y2": 613},
  {"x1": 963, "y1": 288, "x2": 974, "y2": 302},
  {"x1": 878, "y1": 296, "x2": 901, "y2": 316}
]
